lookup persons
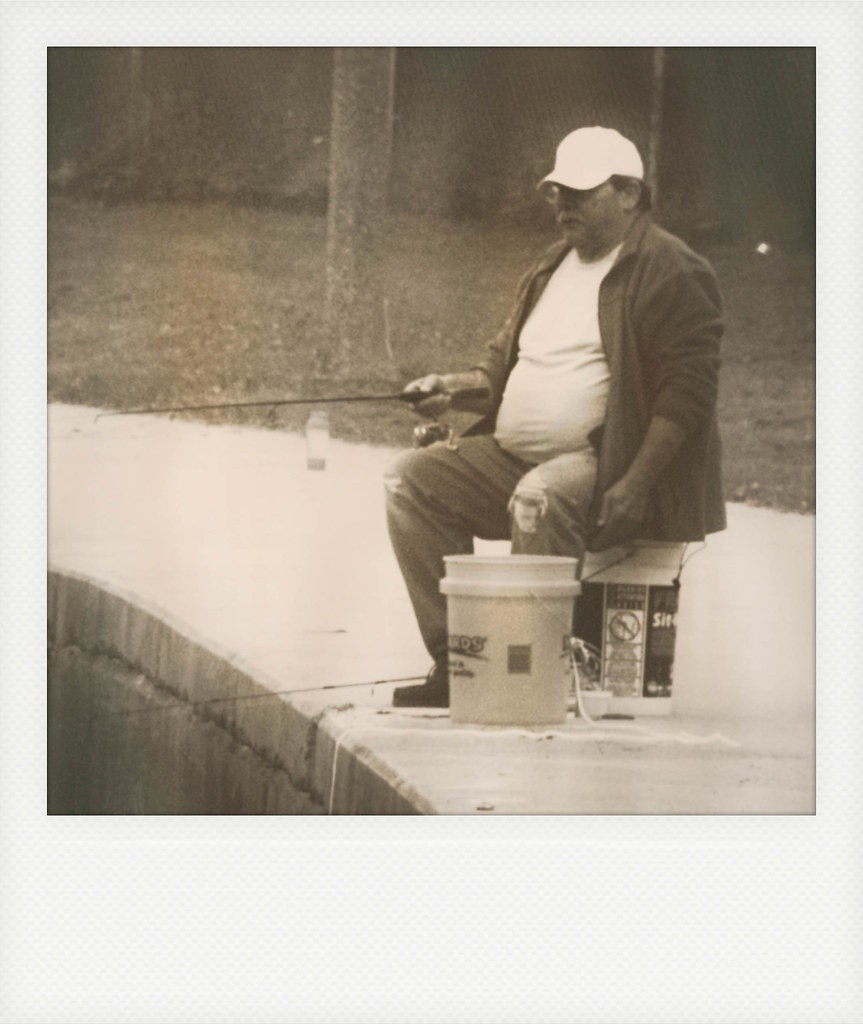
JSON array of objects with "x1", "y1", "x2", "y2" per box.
[{"x1": 386, "y1": 127, "x2": 730, "y2": 718}]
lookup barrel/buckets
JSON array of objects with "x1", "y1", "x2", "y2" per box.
[
  {"x1": 440, "y1": 553, "x2": 583, "y2": 727},
  {"x1": 571, "y1": 540, "x2": 694, "y2": 718}
]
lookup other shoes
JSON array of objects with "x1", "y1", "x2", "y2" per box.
[{"x1": 394, "y1": 666, "x2": 450, "y2": 710}]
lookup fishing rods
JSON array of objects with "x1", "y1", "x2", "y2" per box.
[{"x1": 87, "y1": 384, "x2": 492, "y2": 440}]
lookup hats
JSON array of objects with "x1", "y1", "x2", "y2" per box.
[{"x1": 537, "y1": 124, "x2": 645, "y2": 192}]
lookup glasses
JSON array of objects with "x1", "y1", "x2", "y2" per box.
[{"x1": 539, "y1": 180, "x2": 597, "y2": 203}]
